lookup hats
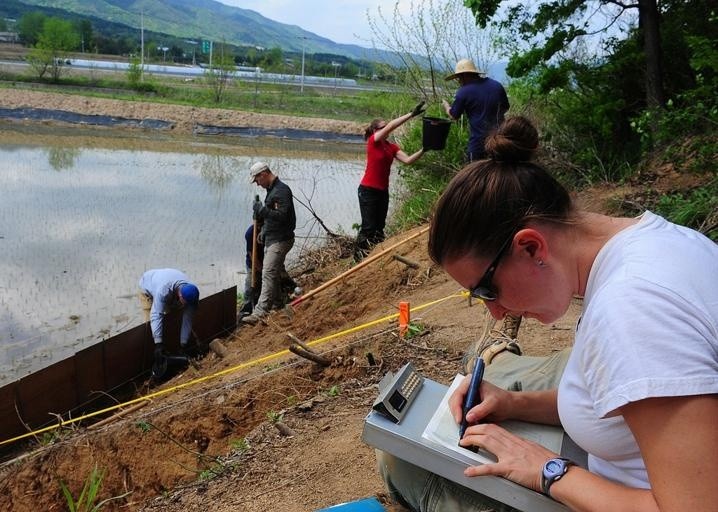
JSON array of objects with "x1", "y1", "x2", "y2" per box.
[
  {"x1": 250, "y1": 162, "x2": 268, "y2": 184},
  {"x1": 181, "y1": 285, "x2": 199, "y2": 310},
  {"x1": 445, "y1": 60, "x2": 486, "y2": 81}
]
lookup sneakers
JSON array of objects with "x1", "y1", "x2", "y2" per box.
[
  {"x1": 242, "y1": 314, "x2": 267, "y2": 327},
  {"x1": 463, "y1": 310, "x2": 522, "y2": 374}
]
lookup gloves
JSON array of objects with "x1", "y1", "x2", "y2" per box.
[
  {"x1": 154, "y1": 344, "x2": 169, "y2": 362},
  {"x1": 253, "y1": 200, "x2": 263, "y2": 212},
  {"x1": 257, "y1": 232, "x2": 264, "y2": 245},
  {"x1": 413, "y1": 101, "x2": 425, "y2": 116}
]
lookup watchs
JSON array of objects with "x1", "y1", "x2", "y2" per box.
[{"x1": 540, "y1": 454, "x2": 578, "y2": 495}]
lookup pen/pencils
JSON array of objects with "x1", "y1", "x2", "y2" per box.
[{"x1": 460, "y1": 359, "x2": 485, "y2": 440}]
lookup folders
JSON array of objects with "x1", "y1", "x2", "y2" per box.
[{"x1": 360, "y1": 369, "x2": 588, "y2": 512}]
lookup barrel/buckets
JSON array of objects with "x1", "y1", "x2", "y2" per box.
[{"x1": 422, "y1": 101, "x2": 453, "y2": 151}]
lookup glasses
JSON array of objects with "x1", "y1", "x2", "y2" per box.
[
  {"x1": 471, "y1": 224, "x2": 521, "y2": 301},
  {"x1": 377, "y1": 126, "x2": 385, "y2": 129}
]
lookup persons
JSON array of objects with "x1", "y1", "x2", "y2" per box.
[
  {"x1": 377, "y1": 114, "x2": 718, "y2": 511},
  {"x1": 239, "y1": 215, "x2": 306, "y2": 316},
  {"x1": 241, "y1": 160, "x2": 297, "y2": 325},
  {"x1": 137, "y1": 266, "x2": 200, "y2": 365},
  {"x1": 440, "y1": 57, "x2": 511, "y2": 164},
  {"x1": 353, "y1": 100, "x2": 431, "y2": 262}
]
